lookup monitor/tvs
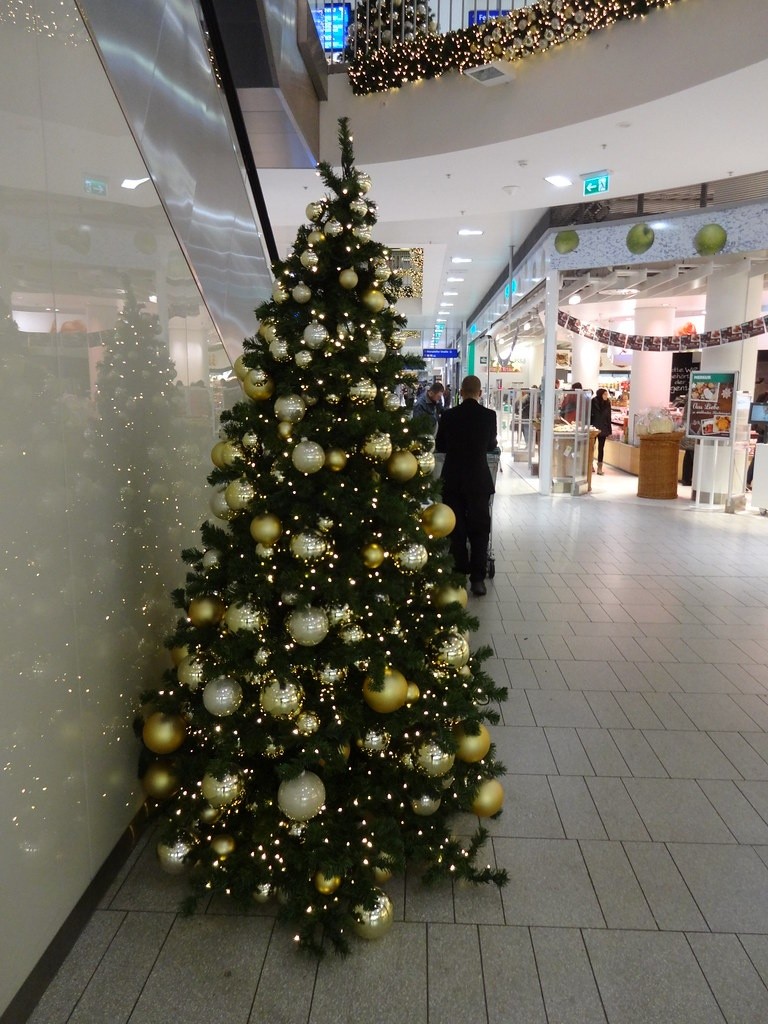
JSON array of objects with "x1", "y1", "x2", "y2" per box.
[{"x1": 748, "y1": 403, "x2": 768, "y2": 424}]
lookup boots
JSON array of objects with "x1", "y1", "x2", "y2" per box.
[{"x1": 597, "y1": 462, "x2": 604, "y2": 475}]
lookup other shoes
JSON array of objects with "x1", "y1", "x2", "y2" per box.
[{"x1": 470, "y1": 576, "x2": 487, "y2": 594}]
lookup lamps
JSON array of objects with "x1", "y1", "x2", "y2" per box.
[
  {"x1": 523, "y1": 323, "x2": 532, "y2": 332},
  {"x1": 598, "y1": 288, "x2": 639, "y2": 296},
  {"x1": 568, "y1": 294, "x2": 581, "y2": 306},
  {"x1": 542, "y1": 175, "x2": 574, "y2": 189},
  {"x1": 462, "y1": 61, "x2": 515, "y2": 88},
  {"x1": 429, "y1": 229, "x2": 484, "y2": 350}
]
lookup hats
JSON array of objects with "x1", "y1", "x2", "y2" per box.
[{"x1": 596, "y1": 389, "x2": 607, "y2": 397}]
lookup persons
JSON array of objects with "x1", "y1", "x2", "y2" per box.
[
  {"x1": 585, "y1": 388, "x2": 614, "y2": 476},
  {"x1": 410, "y1": 382, "x2": 444, "y2": 447},
  {"x1": 559, "y1": 381, "x2": 584, "y2": 426},
  {"x1": 402, "y1": 376, "x2": 428, "y2": 415},
  {"x1": 680, "y1": 446, "x2": 695, "y2": 487},
  {"x1": 432, "y1": 374, "x2": 501, "y2": 596},
  {"x1": 741, "y1": 390, "x2": 768, "y2": 492},
  {"x1": 169, "y1": 365, "x2": 236, "y2": 437},
  {"x1": 511, "y1": 379, "x2": 560, "y2": 459},
  {"x1": 443, "y1": 383, "x2": 452, "y2": 410}
]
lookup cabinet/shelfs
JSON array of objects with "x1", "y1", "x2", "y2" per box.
[{"x1": 611, "y1": 406, "x2": 629, "y2": 442}]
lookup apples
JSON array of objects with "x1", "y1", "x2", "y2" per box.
[
  {"x1": 554, "y1": 230, "x2": 579, "y2": 255},
  {"x1": 693, "y1": 223, "x2": 727, "y2": 256},
  {"x1": 625, "y1": 223, "x2": 654, "y2": 254}
]
